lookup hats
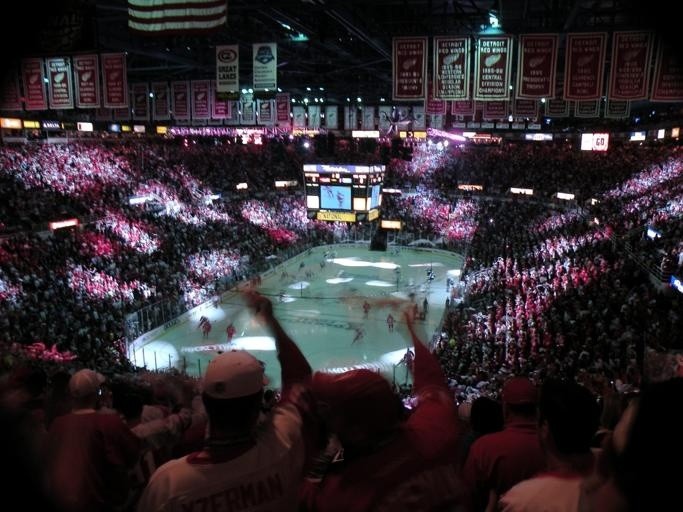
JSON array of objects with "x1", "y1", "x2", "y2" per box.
[
  {"x1": 314, "y1": 370, "x2": 393, "y2": 401},
  {"x1": 68, "y1": 368, "x2": 106, "y2": 397},
  {"x1": 204, "y1": 352, "x2": 269, "y2": 398},
  {"x1": 504, "y1": 380, "x2": 537, "y2": 404}
]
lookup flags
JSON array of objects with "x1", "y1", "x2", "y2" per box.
[{"x1": 124, "y1": 0, "x2": 228, "y2": 33}]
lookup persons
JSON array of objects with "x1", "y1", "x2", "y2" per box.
[
  {"x1": 296, "y1": 260, "x2": 305, "y2": 272},
  {"x1": 294, "y1": 304, "x2": 460, "y2": 512},
  {"x1": 0, "y1": 260, "x2": 273, "y2": 511},
  {"x1": 419, "y1": 260, "x2": 682, "y2": 512},
  {"x1": 362, "y1": 300, "x2": 370, "y2": 320},
  {"x1": 389, "y1": 380, "x2": 418, "y2": 412},
  {"x1": 386, "y1": 313, "x2": 395, "y2": 334},
  {"x1": 406, "y1": 292, "x2": 416, "y2": 303},
  {"x1": 1, "y1": 116, "x2": 683, "y2": 260},
  {"x1": 134, "y1": 286, "x2": 315, "y2": 511},
  {"x1": 277, "y1": 270, "x2": 289, "y2": 283},
  {"x1": 393, "y1": 265, "x2": 401, "y2": 276},
  {"x1": 400, "y1": 347, "x2": 415, "y2": 373},
  {"x1": 318, "y1": 259, "x2": 326, "y2": 272}
]
